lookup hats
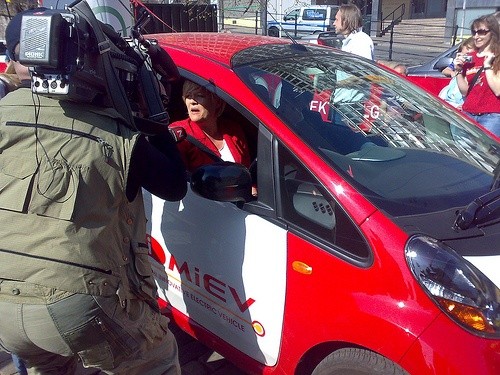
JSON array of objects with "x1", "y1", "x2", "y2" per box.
[{"x1": 6, "y1": 7, "x2": 50, "y2": 62}]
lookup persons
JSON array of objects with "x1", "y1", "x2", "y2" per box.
[
  {"x1": 250, "y1": 70, "x2": 337, "y2": 150},
  {"x1": 167, "y1": 78, "x2": 258, "y2": 208},
  {"x1": 452, "y1": 14, "x2": 500, "y2": 138},
  {"x1": 0, "y1": 0, "x2": 189, "y2": 375},
  {"x1": 394, "y1": 64, "x2": 408, "y2": 78},
  {"x1": 442, "y1": 38, "x2": 476, "y2": 141},
  {"x1": 329, "y1": 3, "x2": 375, "y2": 129}
]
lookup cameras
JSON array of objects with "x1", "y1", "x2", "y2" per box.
[{"x1": 463, "y1": 56, "x2": 473, "y2": 63}]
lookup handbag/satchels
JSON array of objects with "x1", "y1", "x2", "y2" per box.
[{"x1": 445, "y1": 77, "x2": 464, "y2": 104}]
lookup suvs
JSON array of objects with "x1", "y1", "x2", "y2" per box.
[{"x1": 266, "y1": 4, "x2": 366, "y2": 48}]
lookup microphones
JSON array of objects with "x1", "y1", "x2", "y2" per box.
[{"x1": 147, "y1": 43, "x2": 180, "y2": 87}]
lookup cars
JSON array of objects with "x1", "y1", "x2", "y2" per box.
[
  {"x1": 406, "y1": 32, "x2": 479, "y2": 95},
  {"x1": 125, "y1": 31, "x2": 500, "y2": 375}
]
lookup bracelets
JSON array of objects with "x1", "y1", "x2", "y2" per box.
[{"x1": 483, "y1": 67, "x2": 493, "y2": 70}]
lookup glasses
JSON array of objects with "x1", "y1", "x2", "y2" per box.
[{"x1": 471, "y1": 29, "x2": 491, "y2": 36}]
